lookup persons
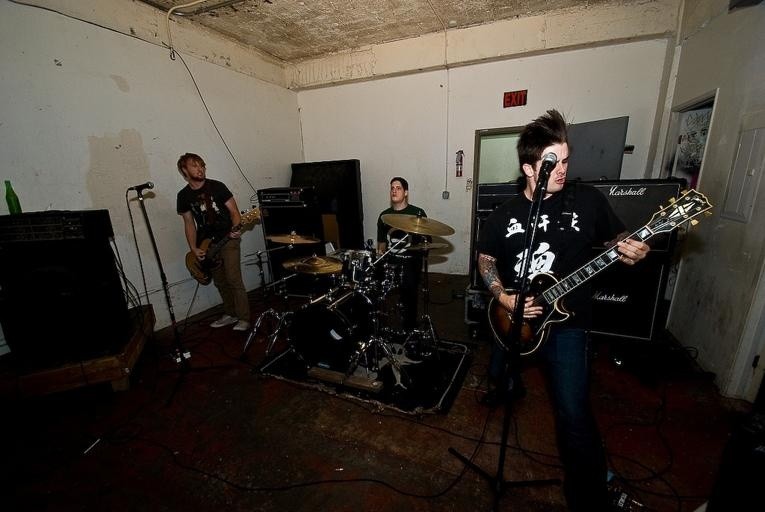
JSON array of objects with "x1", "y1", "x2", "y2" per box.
[
  {"x1": 377, "y1": 176, "x2": 431, "y2": 330},
  {"x1": 475, "y1": 104, "x2": 652, "y2": 512},
  {"x1": 175, "y1": 153, "x2": 254, "y2": 332}
]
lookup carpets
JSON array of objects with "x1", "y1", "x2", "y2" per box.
[{"x1": 256, "y1": 337, "x2": 479, "y2": 416}]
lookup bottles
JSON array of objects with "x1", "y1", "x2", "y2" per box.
[{"x1": 4, "y1": 180, "x2": 22, "y2": 215}]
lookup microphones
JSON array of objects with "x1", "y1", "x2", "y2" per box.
[
  {"x1": 532, "y1": 152, "x2": 557, "y2": 200},
  {"x1": 129, "y1": 182, "x2": 154, "y2": 191}
]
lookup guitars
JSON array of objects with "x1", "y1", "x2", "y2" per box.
[
  {"x1": 184, "y1": 204, "x2": 261, "y2": 285},
  {"x1": 487, "y1": 188, "x2": 713, "y2": 355}
]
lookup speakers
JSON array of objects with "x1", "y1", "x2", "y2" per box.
[
  {"x1": 588, "y1": 177, "x2": 686, "y2": 252},
  {"x1": 578, "y1": 248, "x2": 684, "y2": 343},
  {"x1": 0, "y1": 238, "x2": 133, "y2": 376},
  {"x1": 260, "y1": 205, "x2": 326, "y2": 296}
]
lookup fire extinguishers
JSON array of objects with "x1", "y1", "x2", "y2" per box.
[{"x1": 455, "y1": 150, "x2": 465, "y2": 177}]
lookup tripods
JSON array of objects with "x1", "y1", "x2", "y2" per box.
[
  {"x1": 137, "y1": 195, "x2": 234, "y2": 407},
  {"x1": 448, "y1": 187, "x2": 563, "y2": 512},
  {"x1": 242, "y1": 257, "x2": 443, "y2": 391}
]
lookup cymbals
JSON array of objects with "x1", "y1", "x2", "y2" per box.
[
  {"x1": 406, "y1": 243, "x2": 449, "y2": 250},
  {"x1": 282, "y1": 257, "x2": 344, "y2": 273},
  {"x1": 265, "y1": 236, "x2": 321, "y2": 244},
  {"x1": 381, "y1": 213, "x2": 454, "y2": 236}
]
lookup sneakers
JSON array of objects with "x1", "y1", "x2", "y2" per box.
[
  {"x1": 233, "y1": 319, "x2": 249, "y2": 330},
  {"x1": 211, "y1": 316, "x2": 239, "y2": 328},
  {"x1": 566, "y1": 486, "x2": 645, "y2": 512}
]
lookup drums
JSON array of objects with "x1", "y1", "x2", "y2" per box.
[
  {"x1": 341, "y1": 249, "x2": 388, "y2": 288},
  {"x1": 288, "y1": 287, "x2": 378, "y2": 373},
  {"x1": 387, "y1": 264, "x2": 404, "y2": 289}
]
